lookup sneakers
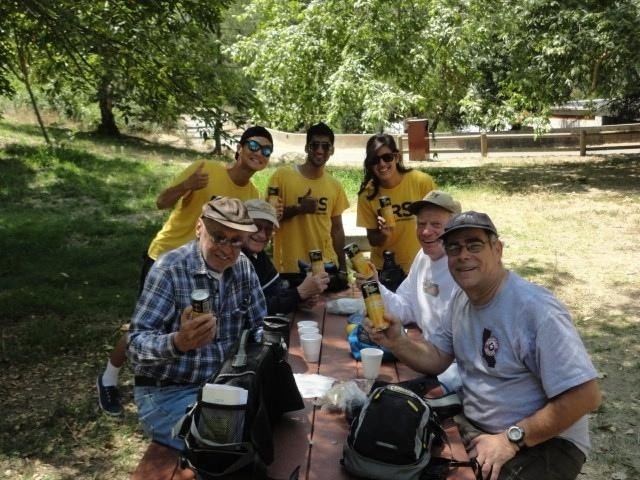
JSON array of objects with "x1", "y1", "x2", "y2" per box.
[{"x1": 96, "y1": 374, "x2": 124, "y2": 416}]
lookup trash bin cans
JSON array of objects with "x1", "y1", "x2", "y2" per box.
[{"x1": 406, "y1": 119, "x2": 430, "y2": 161}]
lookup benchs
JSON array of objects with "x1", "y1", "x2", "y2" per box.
[{"x1": 131, "y1": 440, "x2": 196, "y2": 480}]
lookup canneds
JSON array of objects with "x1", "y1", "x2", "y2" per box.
[
  {"x1": 360, "y1": 281, "x2": 390, "y2": 333},
  {"x1": 379, "y1": 196, "x2": 396, "y2": 229},
  {"x1": 309, "y1": 250, "x2": 325, "y2": 276},
  {"x1": 268, "y1": 186, "x2": 279, "y2": 208},
  {"x1": 190, "y1": 289, "x2": 212, "y2": 320},
  {"x1": 343, "y1": 242, "x2": 374, "y2": 281}
]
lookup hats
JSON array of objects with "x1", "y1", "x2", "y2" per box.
[
  {"x1": 202, "y1": 196, "x2": 279, "y2": 232},
  {"x1": 438, "y1": 211, "x2": 497, "y2": 239},
  {"x1": 407, "y1": 190, "x2": 462, "y2": 213}
]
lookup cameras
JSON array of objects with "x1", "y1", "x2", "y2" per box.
[{"x1": 377, "y1": 250, "x2": 404, "y2": 292}]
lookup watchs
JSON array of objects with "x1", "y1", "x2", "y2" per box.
[{"x1": 505, "y1": 424, "x2": 529, "y2": 455}]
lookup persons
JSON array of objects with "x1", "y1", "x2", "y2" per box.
[
  {"x1": 126, "y1": 197, "x2": 287, "y2": 478},
  {"x1": 353, "y1": 188, "x2": 462, "y2": 390},
  {"x1": 361, "y1": 210, "x2": 602, "y2": 480},
  {"x1": 241, "y1": 199, "x2": 330, "y2": 314},
  {"x1": 357, "y1": 134, "x2": 434, "y2": 275},
  {"x1": 265, "y1": 122, "x2": 349, "y2": 286},
  {"x1": 96, "y1": 126, "x2": 273, "y2": 416}
]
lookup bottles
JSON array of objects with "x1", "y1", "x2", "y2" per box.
[{"x1": 381, "y1": 250, "x2": 397, "y2": 292}]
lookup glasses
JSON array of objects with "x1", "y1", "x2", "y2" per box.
[
  {"x1": 244, "y1": 140, "x2": 272, "y2": 157},
  {"x1": 308, "y1": 142, "x2": 331, "y2": 150},
  {"x1": 207, "y1": 231, "x2": 243, "y2": 247},
  {"x1": 370, "y1": 152, "x2": 396, "y2": 165},
  {"x1": 444, "y1": 239, "x2": 488, "y2": 256}
]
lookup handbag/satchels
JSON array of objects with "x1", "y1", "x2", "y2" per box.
[
  {"x1": 172, "y1": 317, "x2": 304, "y2": 480},
  {"x1": 341, "y1": 375, "x2": 465, "y2": 480},
  {"x1": 348, "y1": 321, "x2": 407, "y2": 363}
]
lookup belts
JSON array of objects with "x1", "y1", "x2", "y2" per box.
[{"x1": 135, "y1": 376, "x2": 174, "y2": 386}]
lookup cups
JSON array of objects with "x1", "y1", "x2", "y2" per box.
[
  {"x1": 360, "y1": 347, "x2": 384, "y2": 380},
  {"x1": 297, "y1": 320, "x2": 323, "y2": 362}
]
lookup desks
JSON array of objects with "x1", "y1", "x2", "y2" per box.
[{"x1": 266, "y1": 283, "x2": 477, "y2": 479}]
